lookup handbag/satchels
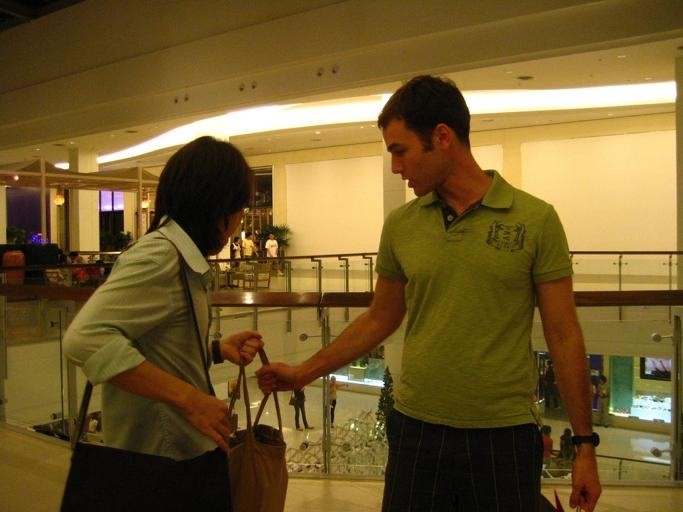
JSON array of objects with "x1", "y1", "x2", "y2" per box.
[
  {"x1": 225, "y1": 341, "x2": 287, "y2": 512},
  {"x1": 59, "y1": 443, "x2": 225, "y2": 512}
]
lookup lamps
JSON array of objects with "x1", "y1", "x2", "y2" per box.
[
  {"x1": 142, "y1": 186, "x2": 150, "y2": 210},
  {"x1": 53, "y1": 185, "x2": 65, "y2": 207}
]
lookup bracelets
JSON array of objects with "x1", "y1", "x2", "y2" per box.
[{"x1": 211, "y1": 339, "x2": 223, "y2": 364}]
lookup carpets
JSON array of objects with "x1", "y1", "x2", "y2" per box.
[{"x1": 5, "y1": 298, "x2": 86, "y2": 347}]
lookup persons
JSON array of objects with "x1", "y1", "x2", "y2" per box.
[
  {"x1": 241, "y1": 232, "x2": 259, "y2": 262},
  {"x1": 292, "y1": 386, "x2": 314, "y2": 431},
  {"x1": 325, "y1": 375, "x2": 350, "y2": 429},
  {"x1": 540, "y1": 425, "x2": 552, "y2": 456},
  {"x1": 254, "y1": 74, "x2": 601, "y2": 511},
  {"x1": 68, "y1": 251, "x2": 88, "y2": 264},
  {"x1": 64, "y1": 136, "x2": 288, "y2": 511},
  {"x1": 557, "y1": 427, "x2": 572, "y2": 458},
  {"x1": 229, "y1": 237, "x2": 241, "y2": 270},
  {"x1": 595, "y1": 373, "x2": 609, "y2": 428},
  {"x1": 264, "y1": 233, "x2": 284, "y2": 276},
  {"x1": 542, "y1": 358, "x2": 560, "y2": 412}
]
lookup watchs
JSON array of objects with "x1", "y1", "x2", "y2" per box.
[{"x1": 570, "y1": 431, "x2": 599, "y2": 447}]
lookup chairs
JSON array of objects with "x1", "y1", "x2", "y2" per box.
[{"x1": 208, "y1": 260, "x2": 273, "y2": 291}]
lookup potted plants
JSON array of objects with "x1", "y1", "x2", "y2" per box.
[{"x1": 255, "y1": 223, "x2": 291, "y2": 270}]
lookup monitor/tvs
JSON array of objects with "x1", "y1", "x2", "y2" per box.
[{"x1": 641, "y1": 357, "x2": 672, "y2": 380}]
